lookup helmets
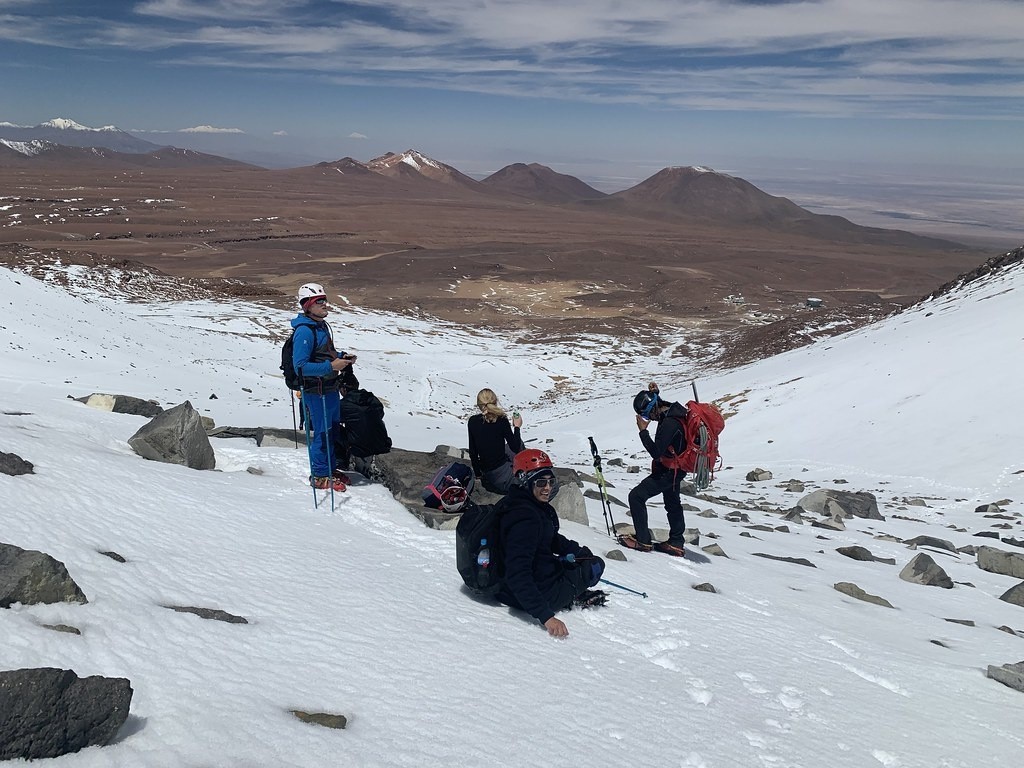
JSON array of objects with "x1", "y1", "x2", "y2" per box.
[
  {"x1": 634, "y1": 391, "x2": 652, "y2": 414},
  {"x1": 297, "y1": 283, "x2": 326, "y2": 303},
  {"x1": 513, "y1": 449, "x2": 553, "y2": 478},
  {"x1": 440, "y1": 486, "x2": 468, "y2": 512},
  {"x1": 517, "y1": 468, "x2": 551, "y2": 486}
]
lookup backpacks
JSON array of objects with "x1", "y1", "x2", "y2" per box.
[
  {"x1": 421, "y1": 462, "x2": 475, "y2": 512},
  {"x1": 456, "y1": 503, "x2": 503, "y2": 596},
  {"x1": 278, "y1": 323, "x2": 317, "y2": 390},
  {"x1": 660, "y1": 400, "x2": 725, "y2": 472}
]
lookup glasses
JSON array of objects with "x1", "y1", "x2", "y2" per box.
[
  {"x1": 534, "y1": 478, "x2": 556, "y2": 487},
  {"x1": 314, "y1": 298, "x2": 327, "y2": 304},
  {"x1": 641, "y1": 393, "x2": 657, "y2": 420}
]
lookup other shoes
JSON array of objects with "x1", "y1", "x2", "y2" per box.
[
  {"x1": 568, "y1": 590, "x2": 611, "y2": 611},
  {"x1": 332, "y1": 470, "x2": 351, "y2": 485},
  {"x1": 653, "y1": 541, "x2": 685, "y2": 557},
  {"x1": 309, "y1": 476, "x2": 346, "y2": 492},
  {"x1": 617, "y1": 534, "x2": 653, "y2": 552}
]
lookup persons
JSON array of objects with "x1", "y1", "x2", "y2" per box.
[
  {"x1": 616, "y1": 390, "x2": 689, "y2": 558},
  {"x1": 333, "y1": 374, "x2": 392, "y2": 473},
  {"x1": 486, "y1": 449, "x2": 605, "y2": 637},
  {"x1": 467, "y1": 388, "x2": 559, "y2": 503},
  {"x1": 291, "y1": 282, "x2": 359, "y2": 492}
]
